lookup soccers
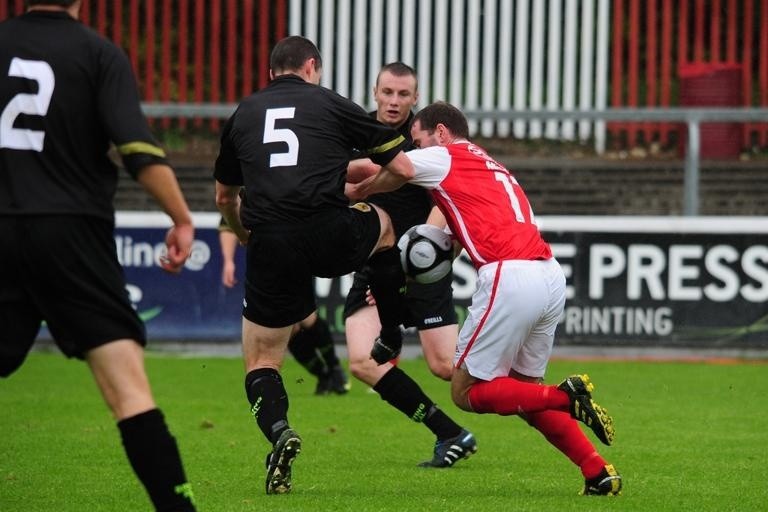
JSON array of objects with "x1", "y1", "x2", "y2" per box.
[{"x1": 397, "y1": 224, "x2": 454, "y2": 284}]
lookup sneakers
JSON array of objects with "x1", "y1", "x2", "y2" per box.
[
  {"x1": 578, "y1": 464, "x2": 623, "y2": 496},
  {"x1": 369, "y1": 336, "x2": 403, "y2": 366},
  {"x1": 416, "y1": 428, "x2": 477, "y2": 468},
  {"x1": 266, "y1": 428, "x2": 303, "y2": 495},
  {"x1": 557, "y1": 374, "x2": 616, "y2": 446},
  {"x1": 316, "y1": 378, "x2": 351, "y2": 396}
]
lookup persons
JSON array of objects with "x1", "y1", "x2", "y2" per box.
[
  {"x1": 340, "y1": 61, "x2": 478, "y2": 468},
  {"x1": 219, "y1": 193, "x2": 348, "y2": 395},
  {"x1": 344, "y1": 99, "x2": 623, "y2": 496},
  {"x1": 1, "y1": 0, "x2": 198, "y2": 512},
  {"x1": 213, "y1": 37, "x2": 416, "y2": 497}
]
